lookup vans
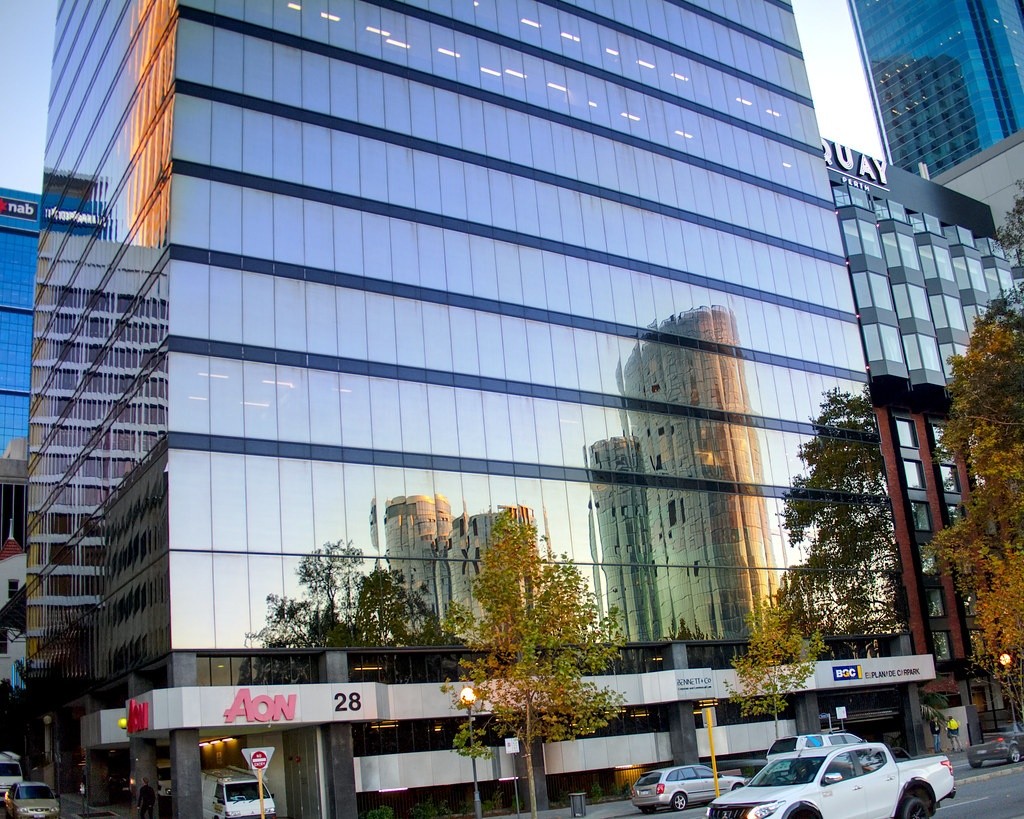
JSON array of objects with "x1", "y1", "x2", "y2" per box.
[
  {"x1": 157, "y1": 758, "x2": 172, "y2": 809},
  {"x1": 0, "y1": 751, "x2": 24, "y2": 805},
  {"x1": 202, "y1": 769, "x2": 276, "y2": 819}
]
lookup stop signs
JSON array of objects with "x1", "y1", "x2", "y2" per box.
[{"x1": 250, "y1": 751, "x2": 268, "y2": 769}]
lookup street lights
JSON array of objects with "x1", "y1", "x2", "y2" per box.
[
  {"x1": 461, "y1": 686, "x2": 484, "y2": 819},
  {"x1": 999, "y1": 652, "x2": 1018, "y2": 733}
]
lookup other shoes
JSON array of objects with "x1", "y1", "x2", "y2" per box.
[
  {"x1": 952, "y1": 748, "x2": 955, "y2": 750},
  {"x1": 960, "y1": 749, "x2": 965, "y2": 752},
  {"x1": 935, "y1": 751, "x2": 942, "y2": 753}
]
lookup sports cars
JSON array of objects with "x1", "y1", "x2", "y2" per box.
[{"x1": 966, "y1": 733, "x2": 1024, "y2": 768}]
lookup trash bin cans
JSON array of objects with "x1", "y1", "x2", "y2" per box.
[{"x1": 570, "y1": 793, "x2": 587, "y2": 817}]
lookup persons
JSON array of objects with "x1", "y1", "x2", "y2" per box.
[
  {"x1": 930, "y1": 715, "x2": 941, "y2": 753},
  {"x1": 945, "y1": 716, "x2": 963, "y2": 753},
  {"x1": 623, "y1": 779, "x2": 631, "y2": 800},
  {"x1": 138, "y1": 778, "x2": 155, "y2": 819}
]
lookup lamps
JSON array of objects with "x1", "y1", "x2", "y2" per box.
[
  {"x1": 118, "y1": 718, "x2": 127, "y2": 729},
  {"x1": 43, "y1": 712, "x2": 53, "y2": 724}
]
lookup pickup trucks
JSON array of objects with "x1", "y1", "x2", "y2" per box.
[{"x1": 703, "y1": 742, "x2": 957, "y2": 819}]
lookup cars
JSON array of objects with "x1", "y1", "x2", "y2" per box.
[
  {"x1": 630, "y1": 765, "x2": 746, "y2": 815},
  {"x1": 767, "y1": 732, "x2": 868, "y2": 762},
  {"x1": 4, "y1": 780, "x2": 60, "y2": 819}
]
